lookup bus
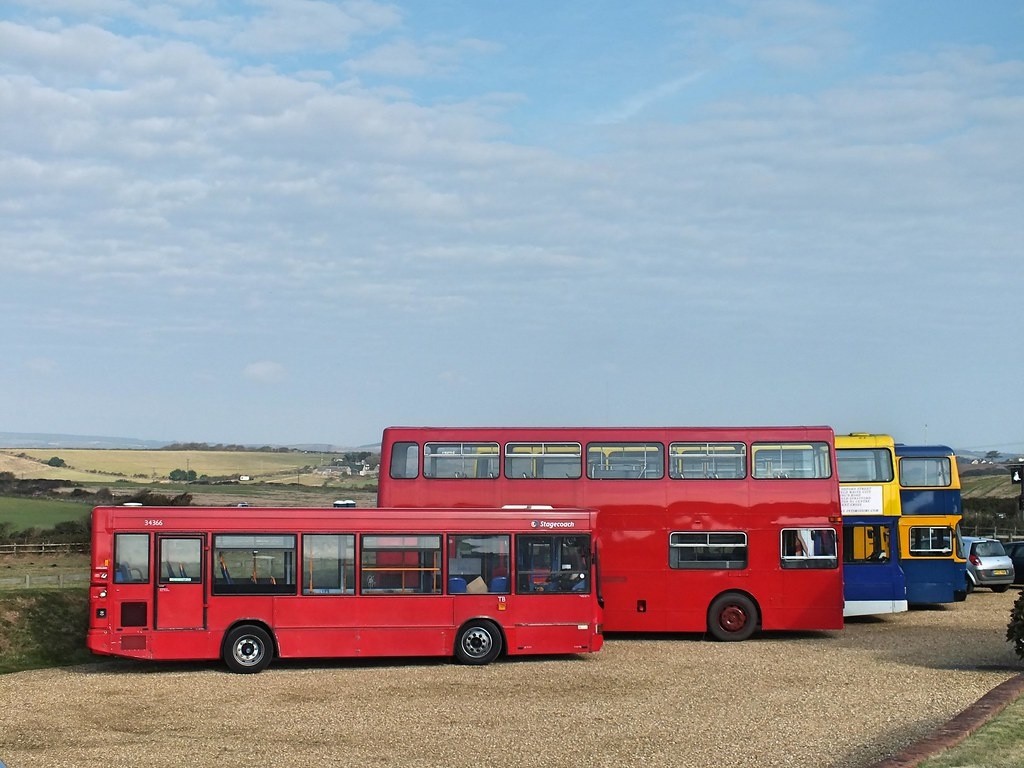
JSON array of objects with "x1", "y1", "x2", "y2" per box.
[
  {"x1": 838, "y1": 445, "x2": 970, "y2": 607},
  {"x1": 373, "y1": 426, "x2": 846, "y2": 643},
  {"x1": 478, "y1": 434, "x2": 909, "y2": 617},
  {"x1": 88, "y1": 498, "x2": 608, "y2": 676}
]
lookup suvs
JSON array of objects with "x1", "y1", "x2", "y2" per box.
[{"x1": 910, "y1": 536, "x2": 1015, "y2": 593}]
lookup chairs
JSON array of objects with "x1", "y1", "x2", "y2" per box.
[
  {"x1": 115, "y1": 552, "x2": 234, "y2": 584},
  {"x1": 448, "y1": 577, "x2": 509, "y2": 593},
  {"x1": 394, "y1": 470, "x2": 790, "y2": 479}
]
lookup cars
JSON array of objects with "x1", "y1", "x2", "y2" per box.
[{"x1": 989, "y1": 542, "x2": 1024, "y2": 584}]
